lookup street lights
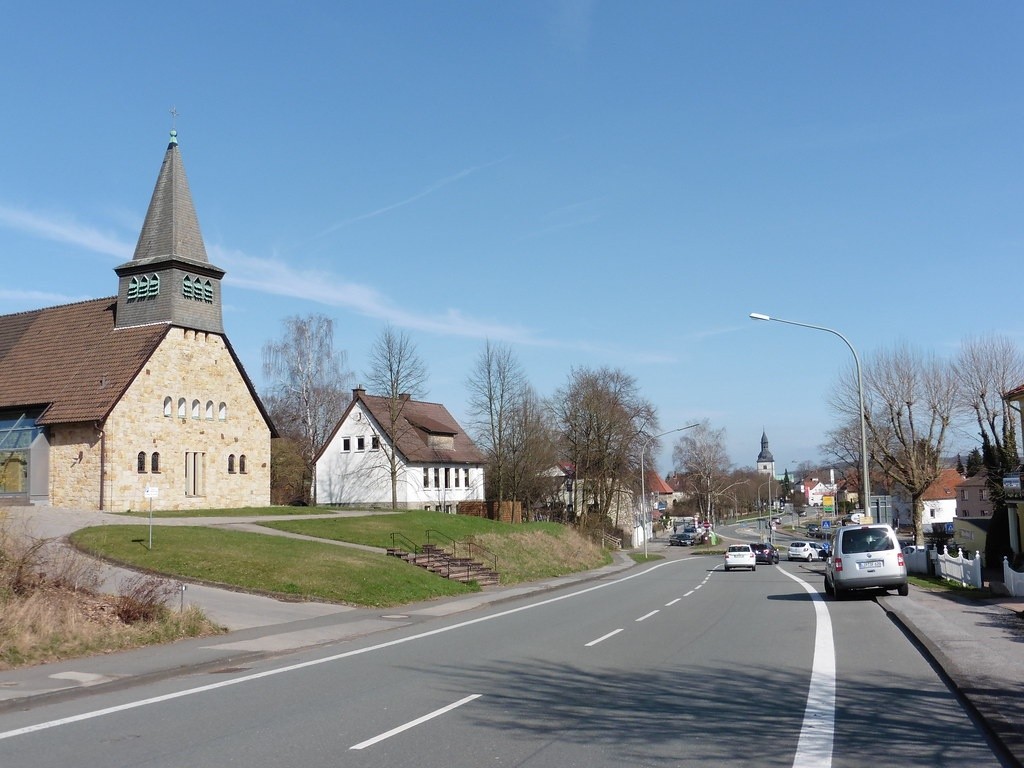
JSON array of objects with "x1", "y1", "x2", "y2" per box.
[
  {"x1": 751, "y1": 313, "x2": 872, "y2": 522},
  {"x1": 641, "y1": 423, "x2": 700, "y2": 560}
]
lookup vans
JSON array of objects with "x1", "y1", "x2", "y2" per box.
[{"x1": 824, "y1": 524, "x2": 908, "y2": 599}]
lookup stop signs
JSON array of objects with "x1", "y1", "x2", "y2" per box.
[{"x1": 703, "y1": 522, "x2": 710, "y2": 528}]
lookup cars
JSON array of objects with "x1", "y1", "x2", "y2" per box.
[
  {"x1": 749, "y1": 542, "x2": 779, "y2": 565},
  {"x1": 787, "y1": 542, "x2": 825, "y2": 562},
  {"x1": 772, "y1": 518, "x2": 782, "y2": 524},
  {"x1": 724, "y1": 545, "x2": 758, "y2": 572},
  {"x1": 670, "y1": 511, "x2": 698, "y2": 547},
  {"x1": 799, "y1": 510, "x2": 807, "y2": 517}
]
